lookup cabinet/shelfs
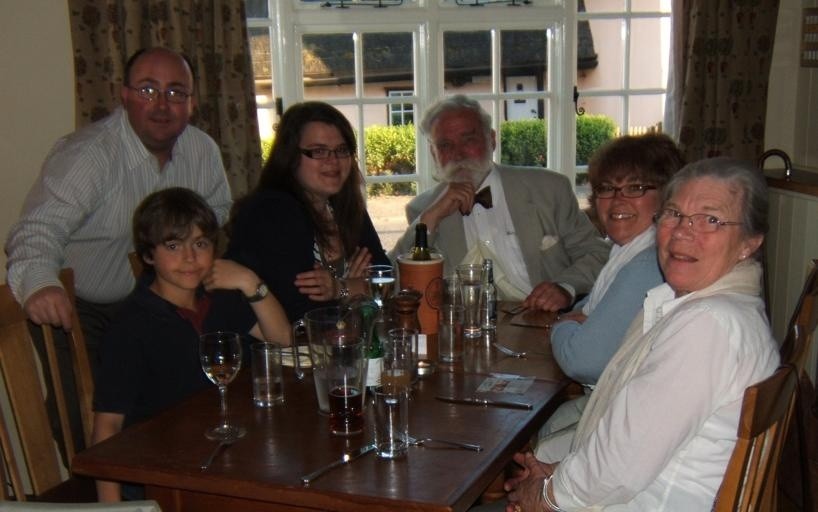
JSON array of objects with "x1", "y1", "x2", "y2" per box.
[{"x1": 765, "y1": 177, "x2": 818, "y2": 348}]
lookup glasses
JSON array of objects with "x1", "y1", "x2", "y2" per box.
[
  {"x1": 299, "y1": 144, "x2": 351, "y2": 161},
  {"x1": 126, "y1": 84, "x2": 194, "y2": 105},
  {"x1": 592, "y1": 182, "x2": 659, "y2": 200},
  {"x1": 652, "y1": 208, "x2": 746, "y2": 235}
]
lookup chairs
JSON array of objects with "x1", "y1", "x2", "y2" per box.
[
  {"x1": 712, "y1": 325, "x2": 812, "y2": 512},
  {"x1": 1, "y1": 267, "x2": 161, "y2": 511},
  {"x1": 779, "y1": 258, "x2": 818, "y2": 361}
]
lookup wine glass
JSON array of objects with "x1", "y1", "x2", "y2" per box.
[{"x1": 199, "y1": 332, "x2": 246, "y2": 441}]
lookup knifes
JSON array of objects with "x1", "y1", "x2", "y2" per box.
[
  {"x1": 299, "y1": 439, "x2": 384, "y2": 484},
  {"x1": 513, "y1": 321, "x2": 553, "y2": 327},
  {"x1": 434, "y1": 395, "x2": 533, "y2": 410}
]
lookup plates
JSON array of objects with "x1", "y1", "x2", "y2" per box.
[{"x1": 275, "y1": 345, "x2": 332, "y2": 368}]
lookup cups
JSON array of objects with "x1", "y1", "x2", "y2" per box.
[
  {"x1": 324, "y1": 335, "x2": 365, "y2": 437},
  {"x1": 390, "y1": 328, "x2": 418, "y2": 391},
  {"x1": 456, "y1": 264, "x2": 482, "y2": 338},
  {"x1": 367, "y1": 265, "x2": 394, "y2": 323},
  {"x1": 250, "y1": 343, "x2": 284, "y2": 408},
  {"x1": 372, "y1": 384, "x2": 408, "y2": 458},
  {"x1": 396, "y1": 253, "x2": 443, "y2": 334},
  {"x1": 439, "y1": 303, "x2": 463, "y2": 363},
  {"x1": 445, "y1": 277, "x2": 461, "y2": 328},
  {"x1": 381, "y1": 339, "x2": 410, "y2": 397}
]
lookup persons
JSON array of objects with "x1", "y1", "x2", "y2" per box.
[
  {"x1": 209, "y1": 100, "x2": 390, "y2": 368},
  {"x1": 480, "y1": 156, "x2": 781, "y2": 511},
  {"x1": 5, "y1": 47, "x2": 233, "y2": 439},
  {"x1": 386, "y1": 95, "x2": 613, "y2": 312},
  {"x1": 78, "y1": 187, "x2": 292, "y2": 501},
  {"x1": 549, "y1": 133, "x2": 687, "y2": 428}
]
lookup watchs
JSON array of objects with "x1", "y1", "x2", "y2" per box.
[
  {"x1": 246, "y1": 282, "x2": 268, "y2": 304},
  {"x1": 337, "y1": 278, "x2": 349, "y2": 300}
]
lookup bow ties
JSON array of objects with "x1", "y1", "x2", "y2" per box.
[{"x1": 459, "y1": 185, "x2": 492, "y2": 216}]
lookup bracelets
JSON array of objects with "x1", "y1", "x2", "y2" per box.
[
  {"x1": 339, "y1": 280, "x2": 350, "y2": 300},
  {"x1": 540, "y1": 474, "x2": 560, "y2": 512}
]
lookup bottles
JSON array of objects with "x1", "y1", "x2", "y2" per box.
[
  {"x1": 359, "y1": 303, "x2": 385, "y2": 395},
  {"x1": 413, "y1": 224, "x2": 430, "y2": 260},
  {"x1": 482, "y1": 259, "x2": 497, "y2": 331}
]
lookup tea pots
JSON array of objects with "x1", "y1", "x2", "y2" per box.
[{"x1": 291, "y1": 307, "x2": 365, "y2": 417}]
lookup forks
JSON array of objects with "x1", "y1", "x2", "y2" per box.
[
  {"x1": 501, "y1": 306, "x2": 527, "y2": 315},
  {"x1": 396, "y1": 425, "x2": 481, "y2": 449}
]
